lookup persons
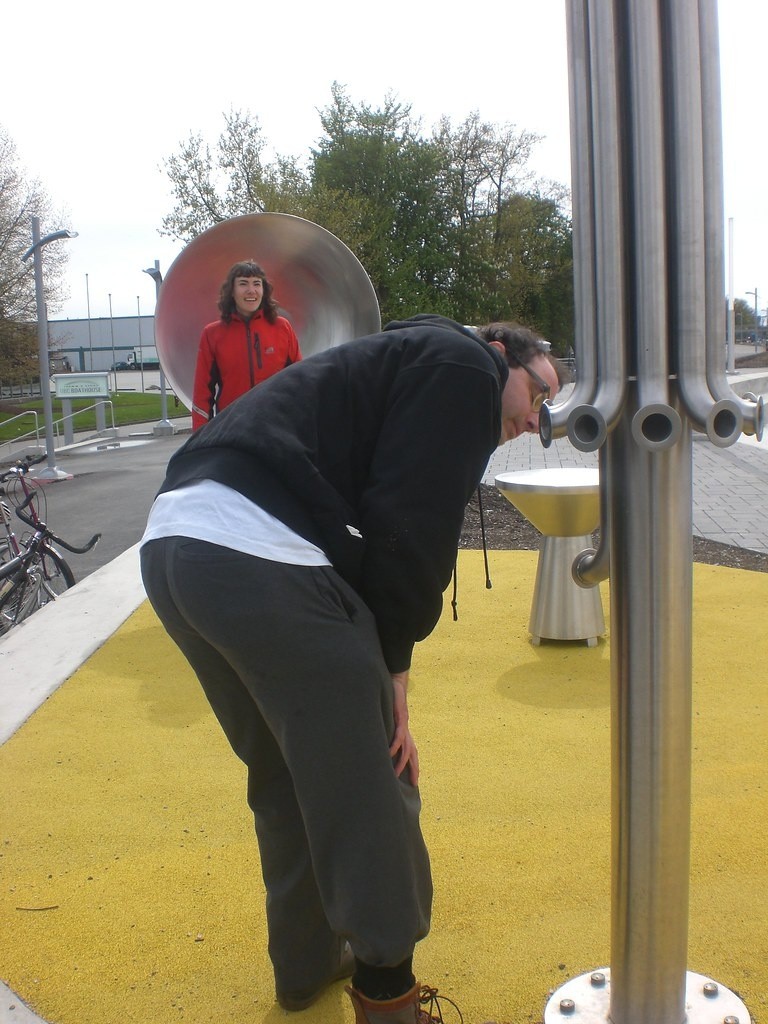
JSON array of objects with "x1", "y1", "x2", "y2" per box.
[
  {"x1": 192, "y1": 261, "x2": 302, "y2": 431},
  {"x1": 139, "y1": 315, "x2": 563, "y2": 1024}
]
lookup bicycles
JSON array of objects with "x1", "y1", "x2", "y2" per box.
[
  {"x1": 0, "y1": 451, "x2": 75, "y2": 626},
  {"x1": 0, "y1": 489, "x2": 103, "y2": 636}
]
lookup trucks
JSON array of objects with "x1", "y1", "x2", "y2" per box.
[{"x1": 127, "y1": 347, "x2": 160, "y2": 371}]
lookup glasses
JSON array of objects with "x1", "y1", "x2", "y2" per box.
[{"x1": 507, "y1": 349, "x2": 551, "y2": 412}]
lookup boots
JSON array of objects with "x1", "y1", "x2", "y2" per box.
[
  {"x1": 344, "y1": 981, "x2": 463, "y2": 1024},
  {"x1": 277, "y1": 940, "x2": 354, "y2": 1010}
]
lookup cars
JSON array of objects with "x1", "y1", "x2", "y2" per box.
[{"x1": 110, "y1": 362, "x2": 129, "y2": 371}]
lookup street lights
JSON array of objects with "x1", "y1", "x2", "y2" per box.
[
  {"x1": 745, "y1": 292, "x2": 758, "y2": 353},
  {"x1": 23, "y1": 229, "x2": 79, "y2": 485}
]
